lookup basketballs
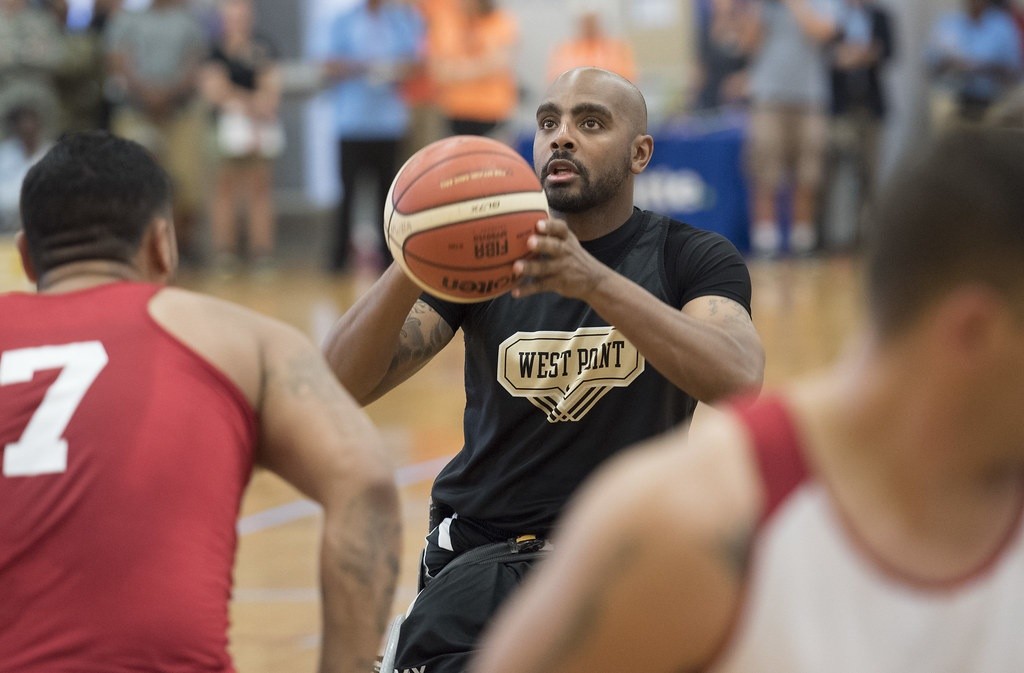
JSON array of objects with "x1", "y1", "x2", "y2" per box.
[{"x1": 384, "y1": 136, "x2": 547, "y2": 306}]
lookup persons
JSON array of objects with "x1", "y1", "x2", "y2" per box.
[
  {"x1": 464, "y1": 92, "x2": 1024, "y2": 673},
  {"x1": 0, "y1": 132, "x2": 404, "y2": 672},
  {"x1": 0, "y1": 0, "x2": 1024, "y2": 278},
  {"x1": 320, "y1": 66, "x2": 764, "y2": 673}
]
